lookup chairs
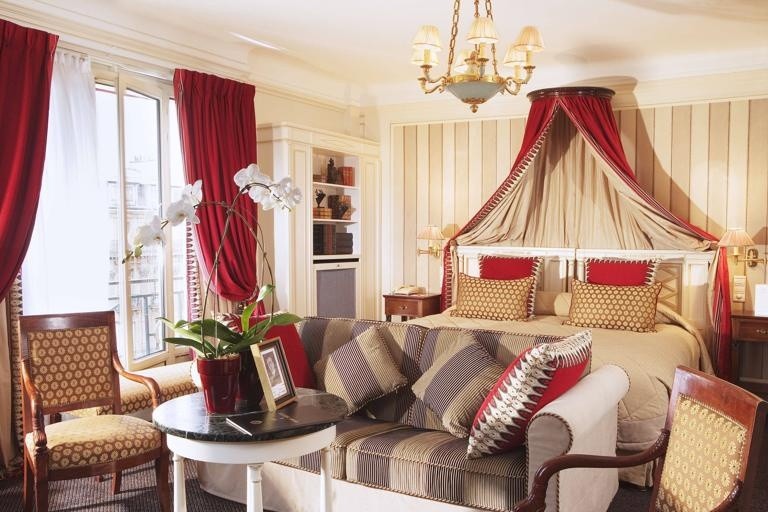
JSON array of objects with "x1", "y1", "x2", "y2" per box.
[
  {"x1": 515, "y1": 365, "x2": 768, "y2": 512},
  {"x1": 18, "y1": 311, "x2": 169, "y2": 512}
]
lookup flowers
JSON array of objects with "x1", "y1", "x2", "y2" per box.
[{"x1": 121, "y1": 162, "x2": 305, "y2": 357}]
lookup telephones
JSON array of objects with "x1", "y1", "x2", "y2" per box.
[{"x1": 390, "y1": 283, "x2": 422, "y2": 296}]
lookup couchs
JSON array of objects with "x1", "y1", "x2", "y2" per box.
[
  {"x1": 51, "y1": 360, "x2": 198, "y2": 485},
  {"x1": 214, "y1": 311, "x2": 627, "y2": 510}
]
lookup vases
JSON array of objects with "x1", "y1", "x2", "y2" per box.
[{"x1": 196, "y1": 353, "x2": 267, "y2": 417}]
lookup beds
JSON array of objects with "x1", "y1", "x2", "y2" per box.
[{"x1": 403, "y1": 246, "x2": 721, "y2": 489}]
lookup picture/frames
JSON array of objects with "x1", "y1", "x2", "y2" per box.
[{"x1": 250, "y1": 337, "x2": 298, "y2": 412}]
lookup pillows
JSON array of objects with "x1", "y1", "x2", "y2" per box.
[
  {"x1": 313, "y1": 326, "x2": 408, "y2": 416},
  {"x1": 411, "y1": 331, "x2": 504, "y2": 438},
  {"x1": 479, "y1": 252, "x2": 542, "y2": 318},
  {"x1": 451, "y1": 272, "x2": 534, "y2": 320},
  {"x1": 210, "y1": 308, "x2": 315, "y2": 389},
  {"x1": 562, "y1": 278, "x2": 663, "y2": 333},
  {"x1": 465, "y1": 330, "x2": 594, "y2": 457},
  {"x1": 582, "y1": 256, "x2": 660, "y2": 288}
]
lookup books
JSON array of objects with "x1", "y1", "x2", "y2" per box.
[
  {"x1": 328, "y1": 195, "x2": 351, "y2": 219},
  {"x1": 337, "y1": 166, "x2": 355, "y2": 186},
  {"x1": 313, "y1": 223, "x2": 353, "y2": 254},
  {"x1": 313, "y1": 174, "x2": 327, "y2": 183}
]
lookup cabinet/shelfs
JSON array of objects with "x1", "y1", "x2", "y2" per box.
[{"x1": 258, "y1": 123, "x2": 380, "y2": 320}]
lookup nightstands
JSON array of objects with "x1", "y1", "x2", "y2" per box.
[
  {"x1": 730, "y1": 310, "x2": 767, "y2": 383},
  {"x1": 383, "y1": 293, "x2": 440, "y2": 322}
]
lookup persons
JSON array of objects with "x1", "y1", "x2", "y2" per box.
[{"x1": 266, "y1": 357, "x2": 282, "y2": 387}]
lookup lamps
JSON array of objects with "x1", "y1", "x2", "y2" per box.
[
  {"x1": 416, "y1": 226, "x2": 445, "y2": 258},
  {"x1": 718, "y1": 228, "x2": 764, "y2": 267},
  {"x1": 411, "y1": 0, "x2": 544, "y2": 114}
]
